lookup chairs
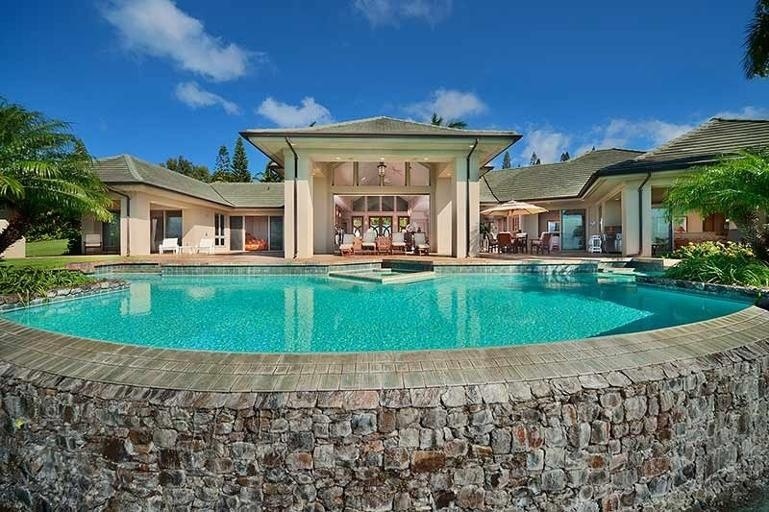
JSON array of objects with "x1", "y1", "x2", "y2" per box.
[
  {"x1": 198, "y1": 237, "x2": 216, "y2": 254},
  {"x1": 337, "y1": 229, "x2": 431, "y2": 256},
  {"x1": 82, "y1": 232, "x2": 103, "y2": 255},
  {"x1": 158, "y1": 236, "x2": 180, "y2": 256},
  {"x1": 481, "y1": 228, "x2": 559, "y2": 257}
]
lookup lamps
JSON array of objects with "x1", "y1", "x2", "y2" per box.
[{"x1": 375, "y1": 160, "x2": 388, "y2": 177}]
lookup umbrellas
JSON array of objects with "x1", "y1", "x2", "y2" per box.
[{"x1": 479, "y1": 200, "x2": 549, "y2": 231}]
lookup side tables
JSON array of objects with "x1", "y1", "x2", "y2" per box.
[{"x1": 180, "y1": 246, "x2": 196, "y2": 255}]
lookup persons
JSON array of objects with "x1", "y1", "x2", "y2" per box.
[{"x1": 515, "y1": 228, "x2": 526, "y2": 254}]
